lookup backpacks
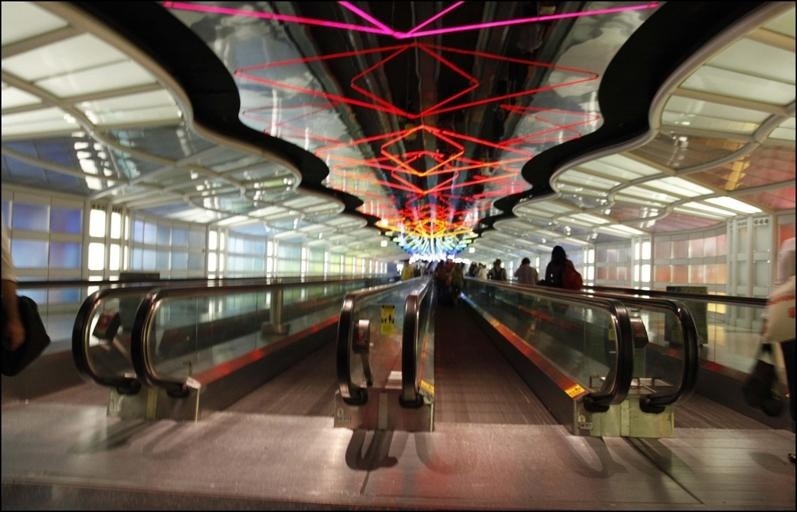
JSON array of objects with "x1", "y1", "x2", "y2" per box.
[
  {"x1": 561, "y1": 260, "x2": 583, "y2": 291},
  {"x1": 494, "y1": 267, "x2": 503, "y2": 280}
]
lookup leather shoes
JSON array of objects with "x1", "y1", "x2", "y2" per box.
[{"x1": 788, "y1": 452, "x2": 796, "y2": 463}]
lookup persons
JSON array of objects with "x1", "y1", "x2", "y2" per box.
[
  {"x1": 0, "y1": 213, "x2": 23, "y2": 352},
  {"x1": 514, "y1": 257, "x2": 538, "y2": 284},
  {"x1": 762, "y1": 221, "x2": 797, "y2": 468},
  {"x1": 544, "y1": 246, "x2": 573, "y2": 288},
  {"x1": 401, "y1": 259, "x2": 506, "y2": 305}
]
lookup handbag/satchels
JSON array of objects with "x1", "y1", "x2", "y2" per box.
[{"x1": 765, "y1": 276, "x2": 796, "y2": 344}]
